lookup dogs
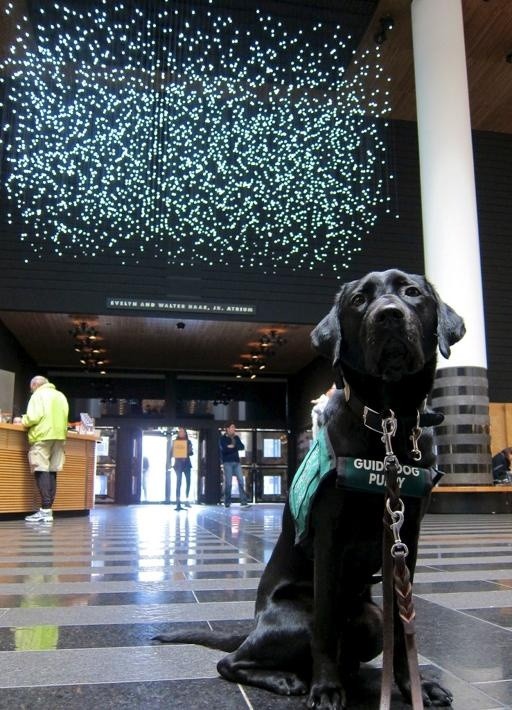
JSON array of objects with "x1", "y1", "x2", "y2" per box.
[{"x1": 148, "y1": 268, "x2": 467, "y2": 710}]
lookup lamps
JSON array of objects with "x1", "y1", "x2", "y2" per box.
[
  {"x1": 73, "y1": 323, "x2": 117, "y2": 404},
  {"x1": 213, "y1": 331, "x2": 288, "y2": 406}
]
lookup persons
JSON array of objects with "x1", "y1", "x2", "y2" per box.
[
  {"x1": 143, "y1": 457, "x2": 149, "y2": 499},
  {"x1": 12, "y1": 376, "x2": 69, "y2": 524},
  {"x1": 491, "y1": 447, "x2": 512, "y2": 485},
  {"x1": 170, "y1": 426, "x2": 193, "y2": 511},
  {"x1": 220, "y1": 422, "x2": 252, "y2": 508}
]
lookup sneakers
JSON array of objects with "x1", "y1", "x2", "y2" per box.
[{"x1": 23, "y1": 507, "x2": 54, "y2": 523}]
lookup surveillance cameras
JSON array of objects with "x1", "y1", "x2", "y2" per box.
[{"x1": 176, "y1": 322, "x2": 186, "y2": 332}]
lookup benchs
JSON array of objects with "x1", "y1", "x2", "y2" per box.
[{"x1": 430, "y1": 485, "x2": 512, "y2": 514}]
